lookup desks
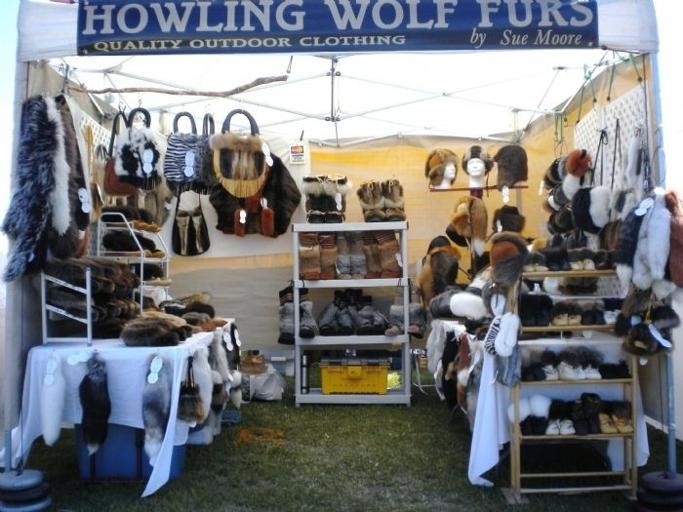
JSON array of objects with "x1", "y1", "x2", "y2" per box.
[{"x1": 27, "y1": 317, "x2": 241, "y2": 487}]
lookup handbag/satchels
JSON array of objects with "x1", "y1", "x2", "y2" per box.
[
  {"x1": 91, "y1": 107, "x2": 274, "y2": 198},
  {"x1": 172, "y1": 184, "x2": 210, "y2": 256}
]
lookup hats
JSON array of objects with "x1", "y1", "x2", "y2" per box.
[
  {"x1": 489, "y1": 231, "x2": 529, "y2": 283},
  {"x1": 426, "y1": 148, "x2": 458, "y2": 186},
  {"x1": 462, "y1": 145, "x2": 493, "y2": 174},
  {"x1": 494, "y1": 145, "x2": 527, "y2": 191},
  {"x1": 541, "y1": 148, "x2": 591, "y2": 247},
  {"x1": 492, "y1": 205, "x2": 524, "y2": 232}
]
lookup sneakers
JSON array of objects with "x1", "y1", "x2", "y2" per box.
[
  {"x1": 507, "y1": 248, "x2": 634, "y2": 435},
  {"x1": 43, "y1": 206, "x2": 215, "y2": 346}
]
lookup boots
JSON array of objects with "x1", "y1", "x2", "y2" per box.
[{"x1": 278, "y1": 176, "x2": 427, "y2": 344}]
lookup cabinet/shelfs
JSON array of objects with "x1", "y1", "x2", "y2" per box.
[
  {"x1": 37, "y1": 211, "x2": 170, "y2": 344},
  {"x1": 290, "y1": 222, "x2": 412, "y2": 409},
  {"x1": 508, "y1": 267, "x2": 639, "y2": 501}
]
